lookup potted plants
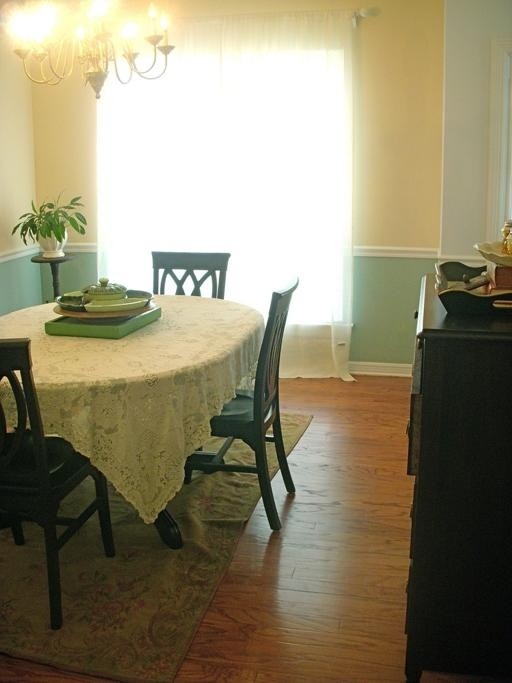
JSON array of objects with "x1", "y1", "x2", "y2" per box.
[{"x1": 18, "y1": 194, "x2": 97, "y2": 255}]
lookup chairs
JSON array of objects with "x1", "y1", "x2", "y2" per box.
[
  {"x1": 1, "y1": 329, "x2": 121, "y2": 637},
  {"x1": 176, "y1": 276, "x2": 304, "y2": 534},
  {"x1": 145, "y1": 249, "x2": 233, "y2": 299}
]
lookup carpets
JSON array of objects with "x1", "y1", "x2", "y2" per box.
[{"x1": 2, "y1": 396, "x2": 318, "y2": 683}]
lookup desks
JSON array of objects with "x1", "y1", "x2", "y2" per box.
[
  {"x1": 0, "y1": 294, "x2": 266, "y2": 556},
  {"x1": 31, "y1": 250, "x2": 75, "y2": 302}
]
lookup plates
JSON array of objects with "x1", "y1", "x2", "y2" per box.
[
  {"x1": 472, "y1": 240, "x2": 512, "y2": 268},
  {"x1": 56, "y1": 288, "x2": 152, "y2": 312},
  {"x1": 53, "y1": 303, "x2": 156, "y2": 324}
]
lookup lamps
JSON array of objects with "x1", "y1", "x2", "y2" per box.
[{"x1": 2, "y1": 0, "x2": 189, "y2": 95}]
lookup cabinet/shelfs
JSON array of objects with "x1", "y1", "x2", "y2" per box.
[{"x1": 396, "y1": 272, "x2": 512, "y2": 681}]
